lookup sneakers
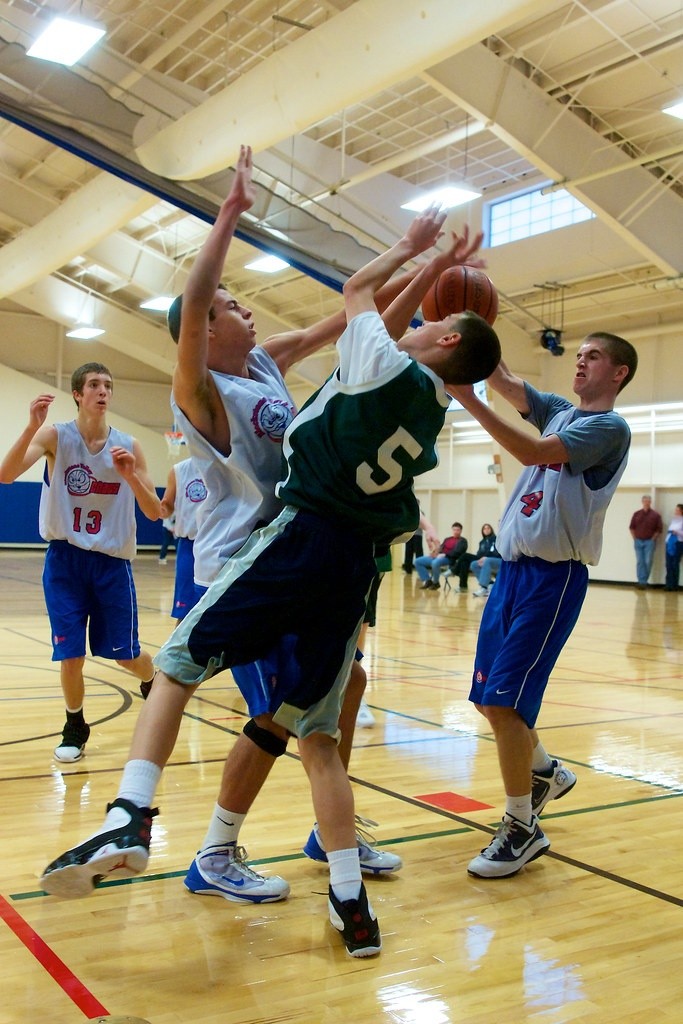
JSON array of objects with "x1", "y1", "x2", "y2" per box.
[
  {"x1": 310, "y1": 880, "x2": 382, "y2": 958},
  {"x1": 303, "y1": 814, "x2": 402, "y2": 875},
  {"x1": 39, "y1": 797, "x2": 160, "y2": 899},
  {"x1": 140, "y1": 671, "x2": 158, "y2": 699},
  {"x1": 354, "y1": 698, "x2": 375, "y2": 728},
  {"x1": 467, "y1": 812, "x2": 551, "y2": 879},
  {"x1": 53, "y1": 722, "x2": 90, "y2": 763},
  {"x1": 502, "y1": 759, "x2": 577, "y2": 822},
  {"x1": 183, "y1": 841, "x2": 291, "y2": 904}
]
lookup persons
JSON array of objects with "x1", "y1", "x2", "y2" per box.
[
  {"x1": 629, "y1": 496, "x2": 663, "y2": 589},
  {"x1": 413, "y1": 522, "x2": 468, "y2": 591},
  {"x1": 442, "y1": 523, "x2": 496, "y2": 593},
  {"x1": 441, "y1": 333, "x2": 638, "y2": 880},
  {"x1": 36, "y1": 142, "x2": 500, "y2": 959},
  {"x1": 401, "y1": 498, "x2": 426, "y2": 574},
  {"x1": 663, "y1": 504, "x2": 683, "y2": 591},
  {"x1": 0, "y1": 362, "x2": 158, "y2": 761},
  {"x1": 470, "y1": 552, "x2": 502, "y2": 598}
]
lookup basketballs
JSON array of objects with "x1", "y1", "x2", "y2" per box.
[{"x1": 421, "y1": 265, "x2": 500, "y2": 328}]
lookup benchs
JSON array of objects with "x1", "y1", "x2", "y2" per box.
[{"x1": 440, "y1": 565, "x2": 496, "y2": 591}]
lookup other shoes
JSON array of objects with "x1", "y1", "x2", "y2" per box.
[
  {"x1": 430, "y1": 583, "x2": 440, "y2": 590},
  {"x1": 441, "y1": 569, "x2": 452, "y2": 577},
  {"x1": 472, "y1": 588, "x2": 487, "y2": 597},
  {"x1": 455, "y1": 587, "x2": 468, "y2": 593},
  {"x1": 420, "y1": 580, "x2": 432, "y2": 589},
  {"x1": 158, "y1": 559, "x2": 167, "y2": 565}
]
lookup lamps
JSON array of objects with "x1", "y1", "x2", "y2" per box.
[
  {"x1": 400, "y1": 114, "x2": 484, "y2": 215},
  {"x1": 65, "y1": 269, "x2": 105, "y2": 339},
  {"x1": 662, "y1": 97, "x2": 683, "y2": 120},
  {"x1": 140, "y1": 217, "x2": 176, "y2": 312},
  {"x1": 24, "y1": 0, "x2": 106, "y2": 67},
  {"x1": 245, "y1": 255, "x2": 289, "y2": 274}
]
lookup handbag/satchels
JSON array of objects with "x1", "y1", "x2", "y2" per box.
[{"x1": 667, "y1": 530, "x2": 677, "y2": 555}]
